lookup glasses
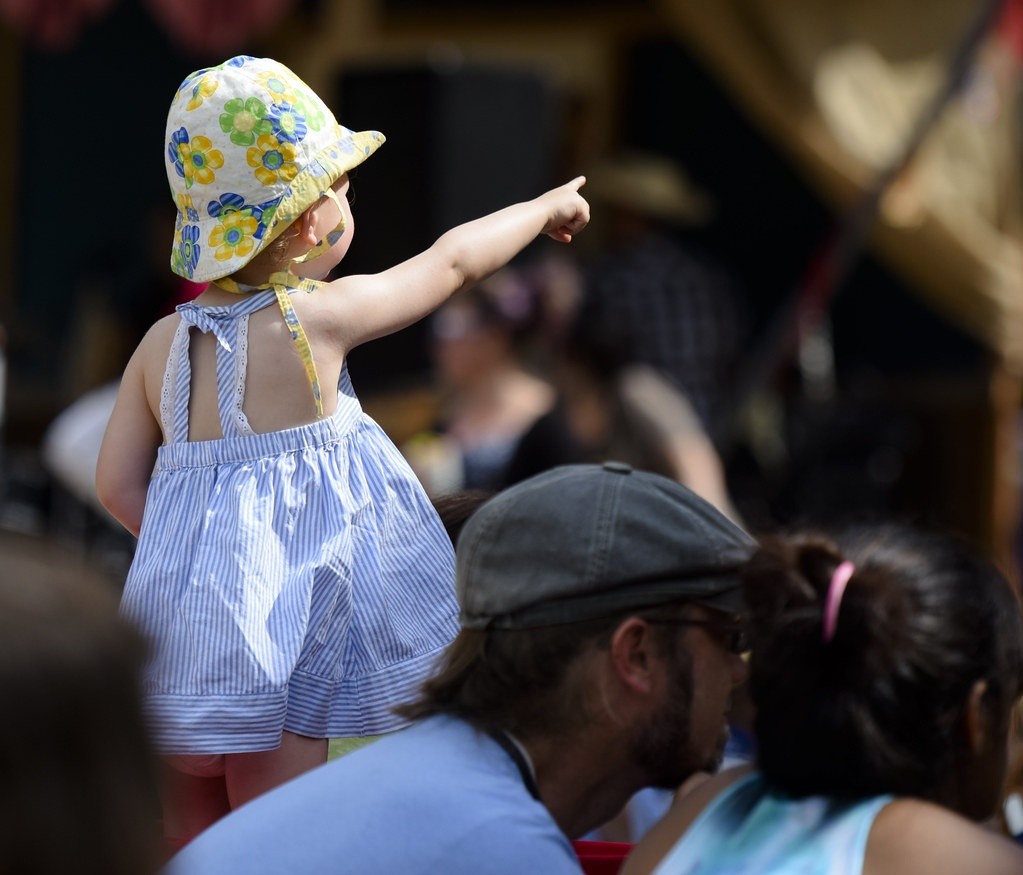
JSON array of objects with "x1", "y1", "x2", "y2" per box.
[{"x1": 593, "y1": 606, "x2": 744, "y2": 658}]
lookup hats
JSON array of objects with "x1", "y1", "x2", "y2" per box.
[
  {"x1": 163, "y1": 56, "x2": 386, "y2": 283},
  {"x1": 456, "y1": 462, "x2": 765, "y2": 630}
]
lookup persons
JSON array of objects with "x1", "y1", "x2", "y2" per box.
[
  {"x1": 151, "y1": 458, "x2": 771, "y2": 874},
  {"x1": 93, "y1": 51, "x2": 594, "y2": 848},
  {"x1": 1, "y1": 204, "x2": 760, "y2": 689},
  {"x1": 649, "y1": 531, "x2": 1023, "y2": 875},
  {"x1": 0, "y1": 540, "x2": 176, "y2": 874}
]
